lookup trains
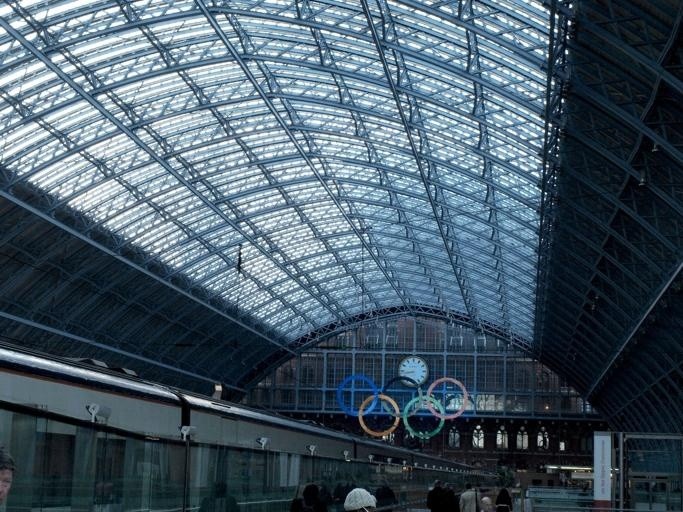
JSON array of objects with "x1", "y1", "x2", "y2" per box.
[{"x1": 0, "y1": 340, "x2": 481, "y2": 512}]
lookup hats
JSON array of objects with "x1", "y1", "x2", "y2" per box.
[{"x1": 342, "y1": 488, "x2": 377, "y2": 511}]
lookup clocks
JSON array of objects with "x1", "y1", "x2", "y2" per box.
[{"x1": 397, "y1": 355, "x2": 430, "y2": 388}]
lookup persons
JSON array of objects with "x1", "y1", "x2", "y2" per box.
[
  {"x1": 293, "y1": 473, "x2": 524, "y2": 512},
  {"x1": 198, "y1": 478, "x2": 239, "y2": 511},
  {"x1": 0, "y1": 444, "x2": 17, "y2": 504}
]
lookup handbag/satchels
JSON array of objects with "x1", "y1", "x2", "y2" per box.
[{"x1": 475, "y1": 503, "x2": 481, "y2": 512}]
